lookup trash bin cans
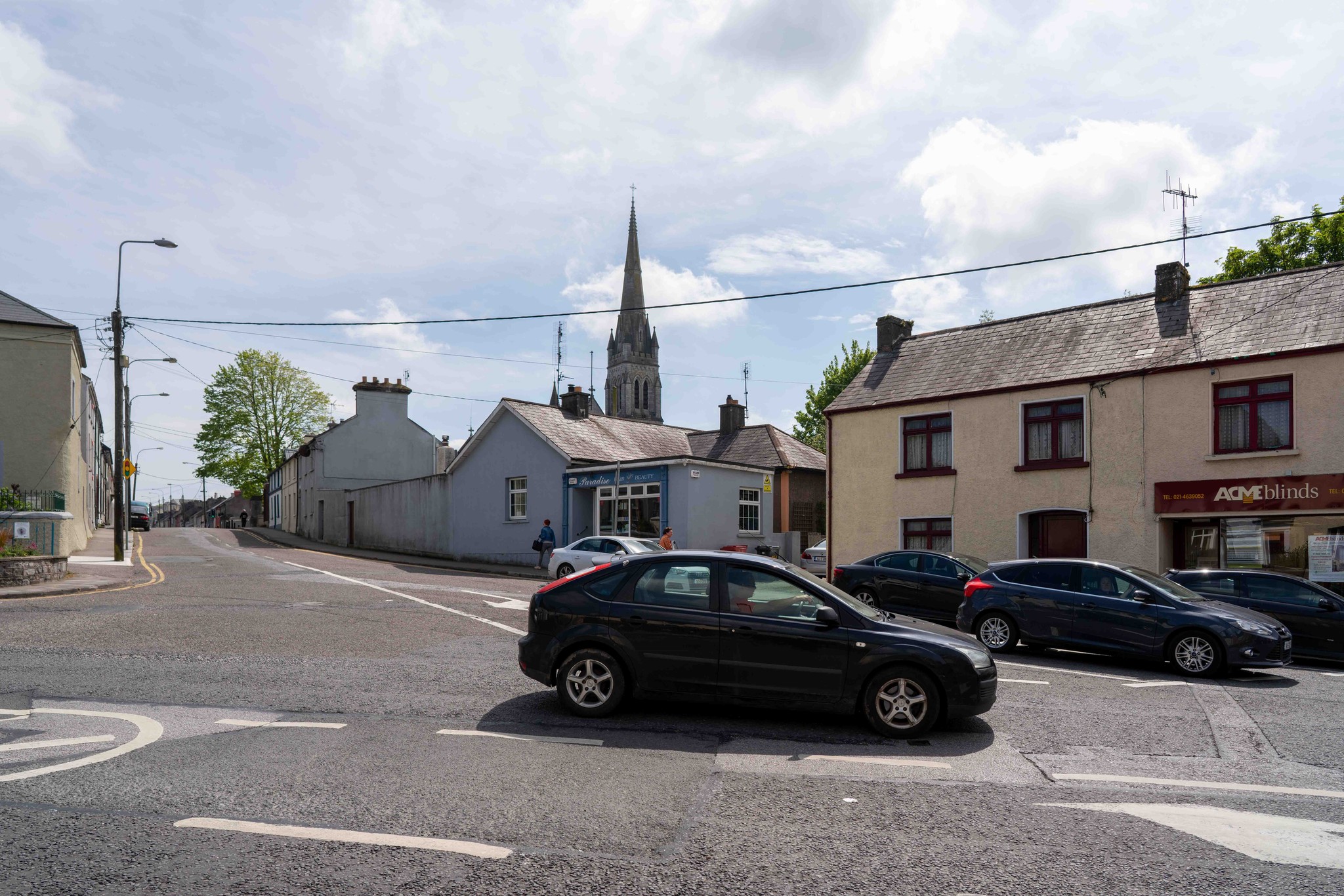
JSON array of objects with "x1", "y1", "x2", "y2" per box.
[
  {"x1": 720, "y1": 545, "x2": 748, "y2": 553},
  {"x1": 754, "y1": 545, "x2": 781, "y2": 560}
]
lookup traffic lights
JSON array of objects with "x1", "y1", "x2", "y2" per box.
[
  {"x1": 124, "y1": 358, "x2": 177, "y2": 530},
  {"x1": 211, "y1": 508, "x2": 216, "y2": 518}
]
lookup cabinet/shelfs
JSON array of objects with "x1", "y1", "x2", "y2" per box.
[{"x1": 601, "y1": 493, "x2": 642, "y2": 538}]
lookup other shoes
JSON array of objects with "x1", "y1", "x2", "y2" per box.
[{"x1": 534, "y1": 566, "x2": 541, "y2": 569}]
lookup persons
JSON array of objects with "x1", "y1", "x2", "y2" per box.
[
  {"x1": 659, "y1": 527, "x2": 672, "y2": 550},
  {"x1": 612, "y1": 545, "x2": 625, "y2": 554},
  {"x1": 1096, "y1": 576, "x2": 1134, "y2": 599},
  {"x1": 730, "y1": 572, "x2": 814, "y2": 614},
  {"x1": 534, "y1": 519, "x2": 556, "y2": 570},
  {"x1": 239, "y1": 509, "x2": 248, "y2": 527}
]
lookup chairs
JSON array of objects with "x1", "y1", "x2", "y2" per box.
[
  {"x1": 924, "y1": 556, "x2": 938, "y2": 575},
  {"x1": 935, "y1": 558, "x2": 953, "y2": 577},
  {"x1": 587, "y1": 545, "x2": 596, "y2": 552},
  {"x1": 892, "y1": 557, "x2": 914, "y2": 571}
]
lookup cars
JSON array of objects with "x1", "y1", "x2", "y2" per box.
[
  {"x1": 801, "y1": 538, "x2": 826, "y2": 579},
  {"x1": 518, "y1": 547, "x2": 998, "y2": 740},
  {"x1": 124, "y1": 501, "x2": 153, "y2": 521},
  {"x1": 548, "y1": 535, "x2": 710, "y2": 595},
  {"x1": 124, "y1": 506, "x2": 151, "y2": 531},
  {"x1": 956, "y1": 555, "x2": 1294, "y2": 678},
  {"x1": 832, "y1": 549, "x2": 989, "y2": 621},
  {"x1": 1162, "y1": 567, "x2": 1344, "y2": 663}
]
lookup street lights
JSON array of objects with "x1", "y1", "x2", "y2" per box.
[
  {"x1": 152, "y1": 489, "x2": 165, "y2": 527},
  {"x1": 149, "y1": 493, "x2": 160, "y2": 527},
  {"x1": 133, "y1": 447, "x2": 164, "y2": 500},
  {"x1": 182, "y1": 462, "x2": 207, "y2": 527},
  {"x1": 167, "y1": 484, "x2": 185, "y2": 527},
  {"x1": 141, "y1": 496, "x2": 151, "y2": 528},
  {"x1": 114, "y1": 240, "x2": 179, "y2": 562}
]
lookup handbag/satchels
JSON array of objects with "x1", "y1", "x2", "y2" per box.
[{"x1": 532, "y1": 535, "x2": 543, "y2": 551}]
